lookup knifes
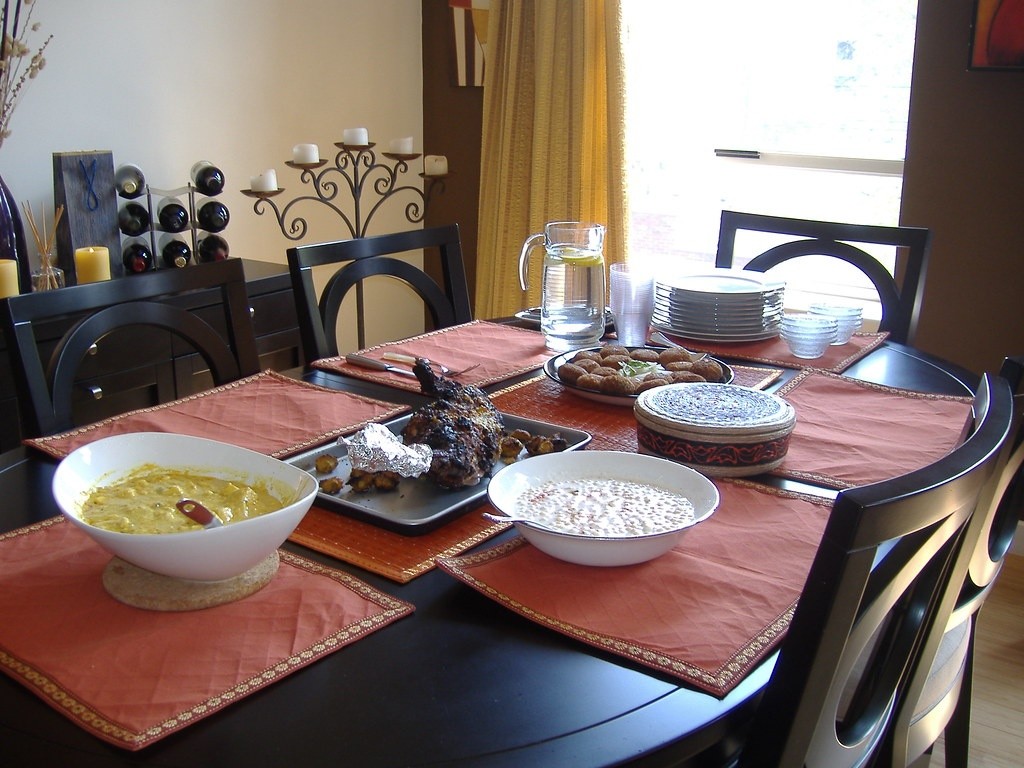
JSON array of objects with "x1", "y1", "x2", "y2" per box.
[{"x1": 345, "y1": 354, "x2": 417, "y2": 378}]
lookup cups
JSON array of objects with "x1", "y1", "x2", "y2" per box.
[{"x1": 609, "y1": 261, "x2": 655, "y2": 346}]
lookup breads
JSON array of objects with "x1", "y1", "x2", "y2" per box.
[{"x1": 558, "y1": 345, "x2": 723, "y2": 395}]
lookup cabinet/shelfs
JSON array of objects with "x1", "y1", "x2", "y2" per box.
[{"x1": 0, "y1": 258, "x2": 303, "y2": 455}]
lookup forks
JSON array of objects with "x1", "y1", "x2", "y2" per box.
[
  {"x1": 650, "y1": 331, "x2": 713, "y2": 361},
  {"x1": 383, "y1": 351, "x2": 481, "y2": 377}
]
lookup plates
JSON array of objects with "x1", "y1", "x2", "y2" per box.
[
  {"x1": 543, "y1": 346, "x2": 735, "y2": 406},
  {"x1": 650, "y1": 269, "x2": 786, "y2": 342},
  {"x1": 514, "y1": 305, "x2": 613, "y2": 327}
]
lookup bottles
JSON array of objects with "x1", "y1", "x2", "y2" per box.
[
  {"x1": 121, "y1": 236, "x2": 152, "y2": 273},
  {"x1": 158, "y1": 233, "x2": 191, "y2": 269},
  {"x1": 114, "y1": 163, "x2": 145, "y2": 198},
  {"x1": 30, "y1": 253, "x2": 65, "y2": 292},
  {"x1": 190, "y1": 160, "x2": 225, "y2": 196},
  {"x1": 195, "y1": 197, "x2": 230, "y2": 233},
  {"x1": 196, "y1": 230, "x2": 229, "y2": 263},
  {"x1": 118, "y1": 201, "x2": 148, "y2": 236},
  {"x1": 157, "y1": 196, "x2": 189, "y2": 233}
]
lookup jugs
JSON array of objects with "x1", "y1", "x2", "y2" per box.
[{"x1": 518, "y1": 222, "x2": 607, "y2": 351}]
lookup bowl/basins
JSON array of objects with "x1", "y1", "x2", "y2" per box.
[
  {"x1": 776, "y1": 303, "x2": 863, "y2": 359},
  {"x1": 487, "y1": 451, "x2": 719, "y2": 566},
  {"x1": 50, "y1": 432, "x2": 317, "y2": 584}
]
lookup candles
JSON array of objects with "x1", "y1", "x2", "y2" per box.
[
  {"x1": 293, "y1": 144, "x2": 319, "y2": 164},
  {"x1": 389, "y1": 136, "x2": 413, "y2": 154},
  {"x1": 75, "y1": 247, "x2": 111, "y2": 285},
  {"x1": 250, "y1": 169, "x2": 278, "y2": 192},
  {"x1": 0, "y1": 260, "x2": 19, "y2": 298},
  {"x1": 425, "y1": 155, "x2": 448, "y2": 175},
  {"x1": 344, "y1": 128, "x2": 368, "y2": 146}
]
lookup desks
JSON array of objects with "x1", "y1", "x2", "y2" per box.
[{"x1": 0, "y1": 308, "x2": 980, "y2": 768}]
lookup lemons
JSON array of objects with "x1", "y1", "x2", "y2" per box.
[{"x1": 561, "y1": 247, "x2": 605, "y2": 267}]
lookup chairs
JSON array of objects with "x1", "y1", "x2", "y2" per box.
[
  {"x1": 286, "y1": 221, "x2": 473, "y2": 365},
  {"x1": 0, "y1": 258, "x2": 259, "y2": 441},
  {"x1": 690, "y1": 355, "x2": 1023, "y2": 768},
  {"x1": 715, "y1": 210, "x2": 931, "y2": 344}
]
